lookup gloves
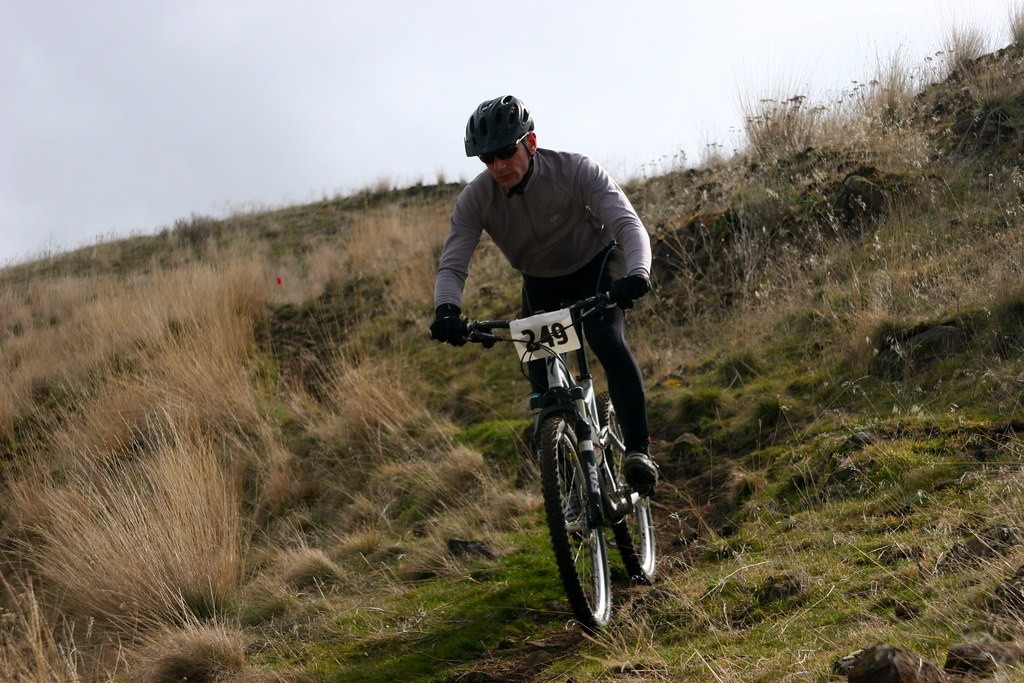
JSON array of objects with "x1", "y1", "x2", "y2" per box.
[
  {"x1": 430, "y1": 313, "x2": 468, "y2": 347},
  {"x1": 613, "y1": 275, "x2": 644, "y2": 309}
]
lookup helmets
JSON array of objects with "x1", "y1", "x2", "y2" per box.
[{"x1": 464, "y1": 95, "x2": 534, "y2": 157}]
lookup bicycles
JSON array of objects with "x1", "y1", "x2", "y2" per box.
[{"x1": 426, "y1": 278, "x2": 659, "y2": 631}]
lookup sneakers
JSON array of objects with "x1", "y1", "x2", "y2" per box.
[
  {"x1": 562, "y1": 490, "x2": 588, "y2": 531},
  {"x1": 623, "y1": 450, "x2": 658, "y2": 494}
]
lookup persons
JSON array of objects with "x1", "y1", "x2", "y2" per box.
[{"x1": 429, "y1": 94, "x2": 660, "y2": 496}]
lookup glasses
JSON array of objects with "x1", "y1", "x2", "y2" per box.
[{"x1": 480, "y1": 132, "x2": 529, "y2": 164}]
